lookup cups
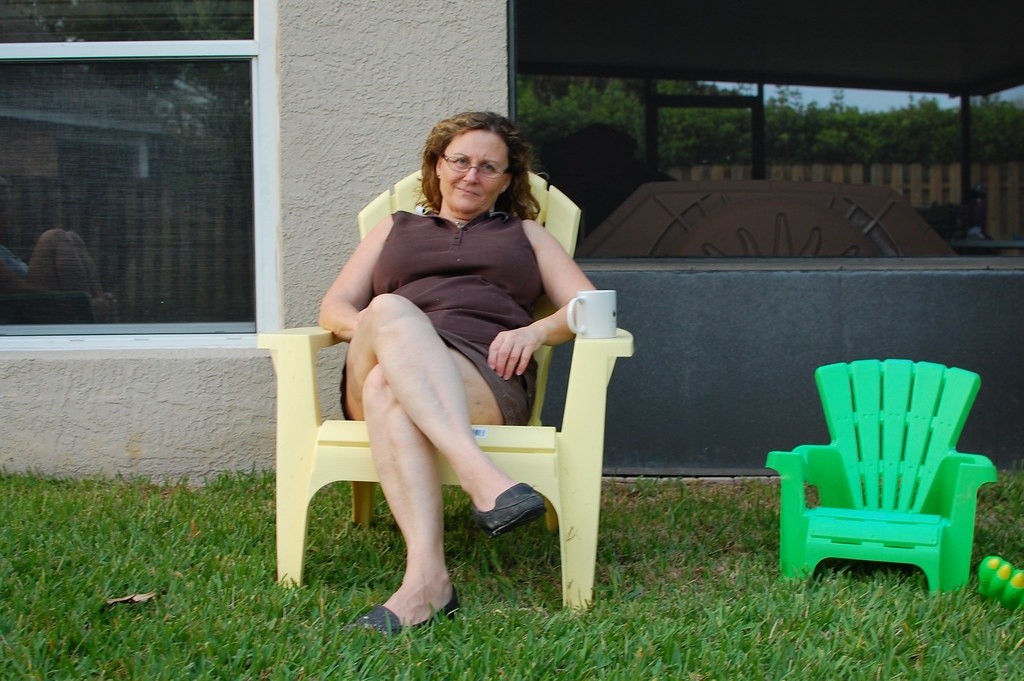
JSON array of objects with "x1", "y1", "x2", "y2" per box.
[{"x1": 567, "y1": 290, "x2": 617, "y2": 338}]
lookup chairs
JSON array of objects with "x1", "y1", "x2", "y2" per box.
[
  {"x1": 764, "y1": 358, "x2": 1002, "y2": 604},
  {"x1": 253, "y1": 165, "x2": 636, "y2": 618}
]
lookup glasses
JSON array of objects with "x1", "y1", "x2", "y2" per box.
[{"x1": 441, "y1": 154, "x2": 510, "y2": 178}]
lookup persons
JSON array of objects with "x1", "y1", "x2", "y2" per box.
[
  {"x1": 317, "y1": 114, "x2": 601, "y2": 631},
  {"x1": 0, "y1": 227, "x2": 121, "y2": 322}
]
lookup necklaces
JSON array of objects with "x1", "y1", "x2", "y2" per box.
[{"x1": 454, "y1": 220, "x2": 466, "y2": 227}]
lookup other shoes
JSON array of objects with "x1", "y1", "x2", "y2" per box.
[
  {"x1": 346, "y1": 583, "x2": 460, "y2": 637},
  {"x1": 473, "y1": 483, "x2": 547, "y2": 538}
]
